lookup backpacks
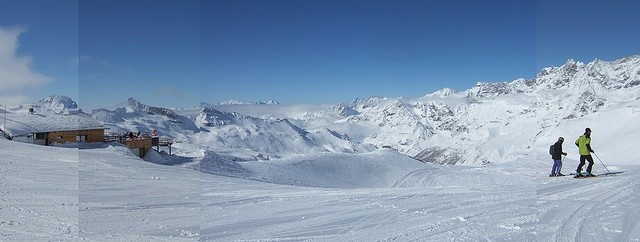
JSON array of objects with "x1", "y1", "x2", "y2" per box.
[{"x1": 550, "y1": 145, "x2": 555, "y2": 155}]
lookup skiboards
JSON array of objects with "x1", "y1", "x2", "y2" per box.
[
  {"x1": 574, "y1": 171, "x2": 623, "y2": 178},
  {"x1": 549, "y1": 172, "x2": 587, "y2": 177}
]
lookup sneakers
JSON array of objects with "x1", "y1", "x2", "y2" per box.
[
  {"x1": 576, "y1": 172, "x2": 581, "y2": 176},
  {"x1": 586, "y1": 172, "x2": 593, "y2": 176}
]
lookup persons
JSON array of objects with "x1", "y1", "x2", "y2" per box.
[
  {"x1": 550, "y1": 137, "x2": 567, "y2": 177},
  {"x1": 575, "y1": 128, "x2": 596, "y2": 178}
]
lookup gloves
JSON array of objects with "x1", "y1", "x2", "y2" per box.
[{"x1": 563, "y1": 152, "x2": 567, "y2": 156}]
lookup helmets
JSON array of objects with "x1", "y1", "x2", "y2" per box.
[{"x1": 559, "y1": 137, "x2": 564, "y2": 142}]
